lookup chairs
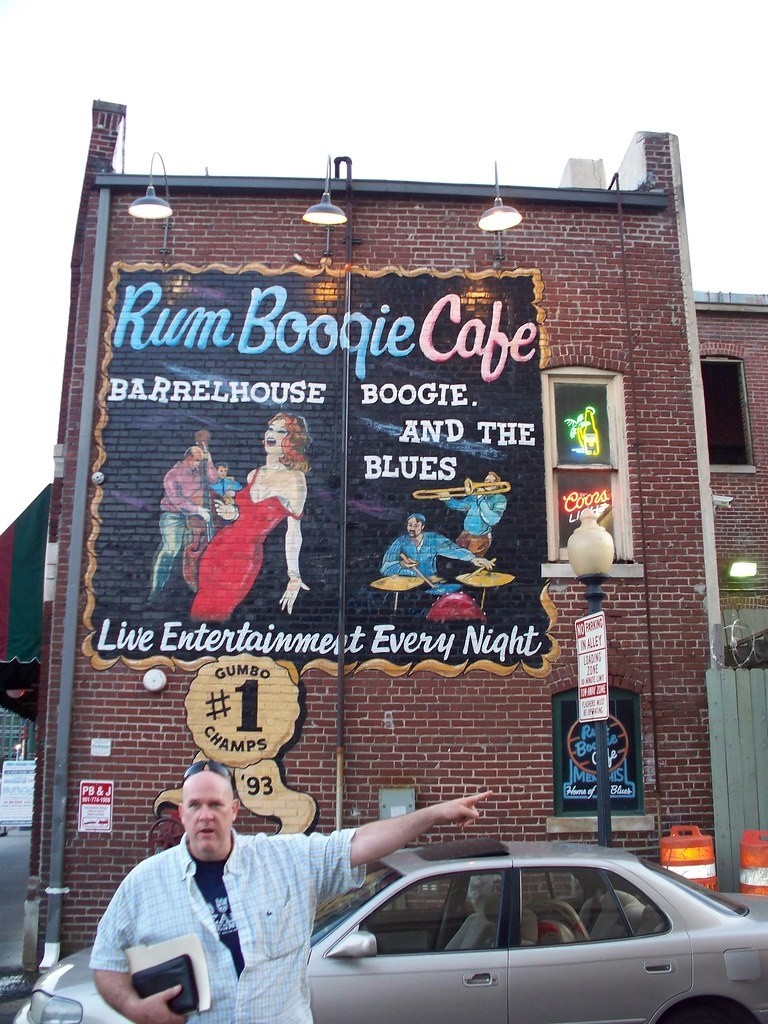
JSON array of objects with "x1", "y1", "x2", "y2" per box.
[{"x1": 445, "y1": 893, "x2": 539, "y2": 949}]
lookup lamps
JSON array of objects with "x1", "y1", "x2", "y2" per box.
[
  {"x1": 726, "y1": 561, "x2": 757, "y2": 581},
  {"x1": 128, "y1": 152, "x2": 173, "y2": 254},
  {"x1": 303, "y1": 154, "x2": 347, "y2": 256},
  {"x1": 478, "y1": 161, "x2": 523, "y2": 261}
]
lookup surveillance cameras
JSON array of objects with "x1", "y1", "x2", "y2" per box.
[{"x1": 713, "y1": 495, "x2": 734, "y2": 508}]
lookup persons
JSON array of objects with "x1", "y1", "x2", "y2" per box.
[{"x1": 87, "y1": 759, "x2": 494, "y2": 1024}]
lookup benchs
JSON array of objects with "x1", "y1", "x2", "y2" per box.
[{"x1": 578, "y1": 889, "x2": 664, "y2": 939}]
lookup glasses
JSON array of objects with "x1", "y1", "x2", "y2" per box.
[{"x1": 182, "y1": 760, "x2": 231, "y2": 787}]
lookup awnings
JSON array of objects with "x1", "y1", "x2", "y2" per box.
[{"x1": 0, "y1": 481, "x2": 51, "y2": 725}]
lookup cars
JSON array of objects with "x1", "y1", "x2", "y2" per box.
[{"x1": 12, "y1": 833, "x2": 768, "y2": 1024}]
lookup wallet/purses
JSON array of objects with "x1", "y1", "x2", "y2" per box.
[{"x1": 132, "y1": 953, "x2": 199, "y2": 1015}]
